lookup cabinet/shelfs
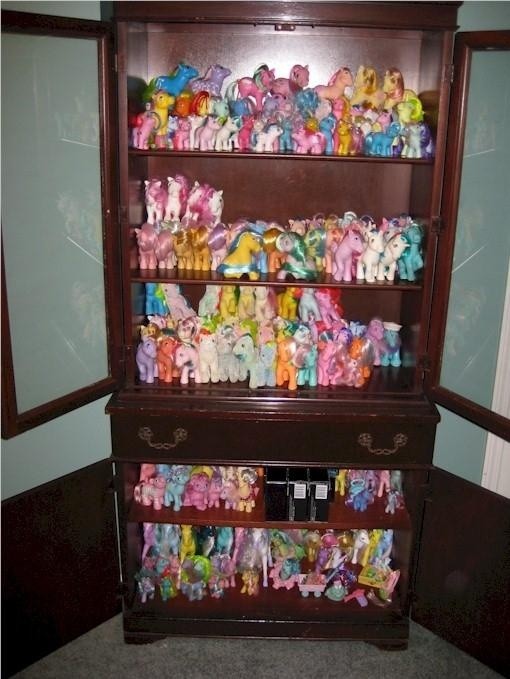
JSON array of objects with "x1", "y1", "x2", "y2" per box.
[
  {"x1": 1, "y1": 390, "x2": 510, "y2": 679},
  {"x1": 2, "y1": 1, "x2": 510, "y2": 445}
]
